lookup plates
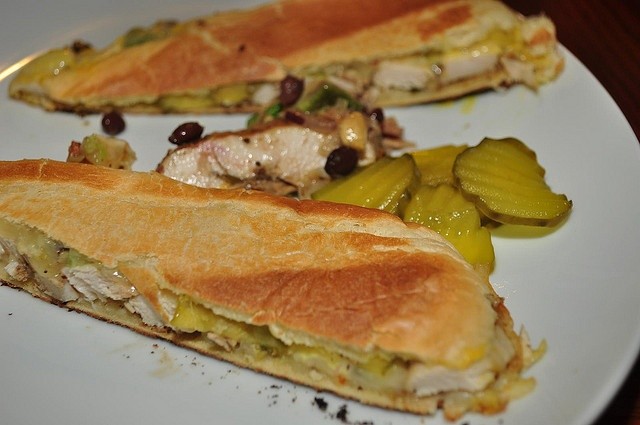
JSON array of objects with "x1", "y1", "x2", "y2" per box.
[{"x1": 0, "y1": 0, "x2": 640, "y2": 425}]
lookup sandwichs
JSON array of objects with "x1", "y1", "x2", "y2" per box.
[
  {"x1": 9, "y1": 1, "x2": 565, "y2": 113},
  {"x1": 1, "y1": 158, "x2": 523, "y2": 418}
]
